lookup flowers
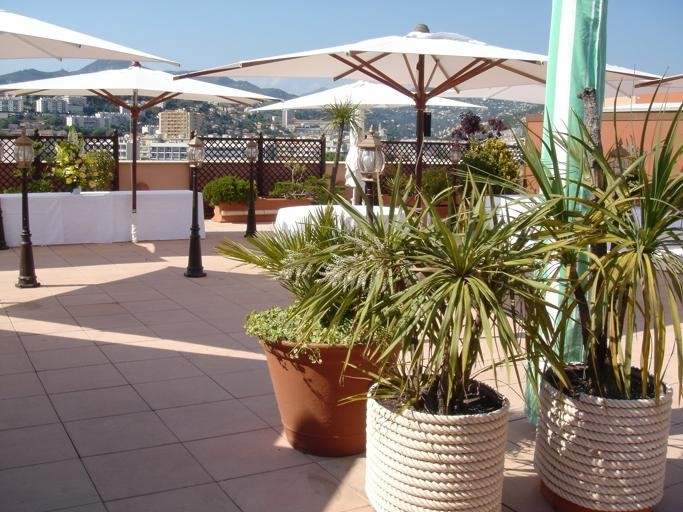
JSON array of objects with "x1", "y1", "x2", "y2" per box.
[{"x1": 62, "y1": 160, "x2": 86, "y2": 186}]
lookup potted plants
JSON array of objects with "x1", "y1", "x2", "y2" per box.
[
  {"x1": 505, "y1": 66, "x2": 683, "y2": 506},
  {"x1": 335, "y1": 135, "x2": 569, "y2": 511},
  {"x1": 213, "y1": 203, "x2": 403, "y2": 456}
]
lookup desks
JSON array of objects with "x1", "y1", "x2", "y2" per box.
[
  {"x1": 0, "y1": 186, "x2": 209, "y2": 248},
  {"x1": 621, "y1": 204, "x2": 683, "y2": 274},
  {"x1": 270, "y1": 205, "x2": 404, "y2": 235},
  {"x1": 477, "y1": 191, "x2": 549, "y2": 233}
]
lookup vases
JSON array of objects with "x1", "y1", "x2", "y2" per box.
[{"x1": 72, "y1": 185, "x2": 81, "y2": 195}]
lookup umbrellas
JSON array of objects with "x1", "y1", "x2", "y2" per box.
[
  {"x1": 345, "y1": 109, "x2": 368, "y2": 206},
  {"x1": 1, "y1": 59, "x2": 285, "y2": 242},
  {"x1": 425, "y1": 81, "x2": 668, "y2": 105},
  {"x1": 630, "y1": 69, "x2": 683, "y2": 96},
  {"x1": 0, "y1": 9, "x2": 182, "y2": 251},
  {"x1": 249, "y1": 80, "x2": 490, "y2": 112},
  {"x1": 173, "y1": 24, "x2": 668, "y2": 213}
]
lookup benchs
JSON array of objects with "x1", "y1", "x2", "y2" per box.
[{"x1": 222, "y1": 208, "x2": 278, "y2": 217}]
